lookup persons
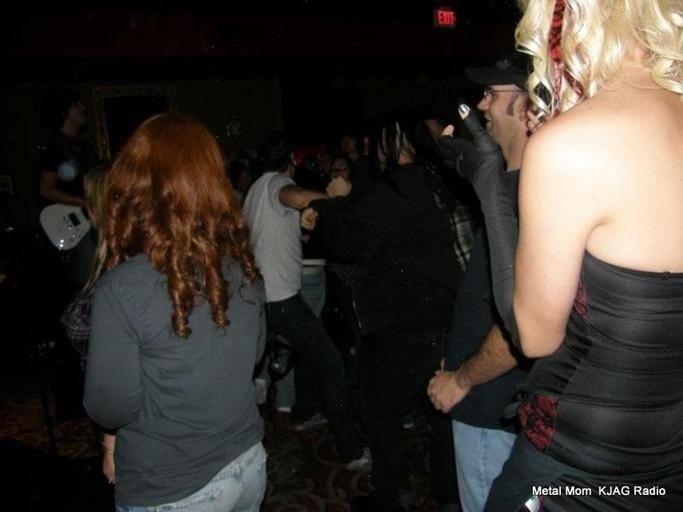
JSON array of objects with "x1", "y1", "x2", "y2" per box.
[{"x1": 0, "y1": 0, "x2": 683, "y2": 510}]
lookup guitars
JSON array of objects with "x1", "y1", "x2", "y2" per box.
[{"x1": 39, "y1": 203, "x2": 93, "y2": 251}]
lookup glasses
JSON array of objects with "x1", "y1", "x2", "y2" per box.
[{"x1": 483, "y1": 87, "x2": 525, "y2": 102}]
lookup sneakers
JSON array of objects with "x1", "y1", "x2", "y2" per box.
[
  {"x1": 271, "y1": 413, "x2": 328, "y2": 440},
  {"x1": 254, "y1": 378, "x2": 267, "y2": 404},
  {"x1": 312, "y1": 436, "x2": 372, "y2": 473}
]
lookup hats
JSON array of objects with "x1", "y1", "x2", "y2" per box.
[
  {"x1": 466, "y1": 54, "x2": 534, "y2": 93},
  {"x1": 267, "y1": 133, "x2": 295, "y2": 172}
]
